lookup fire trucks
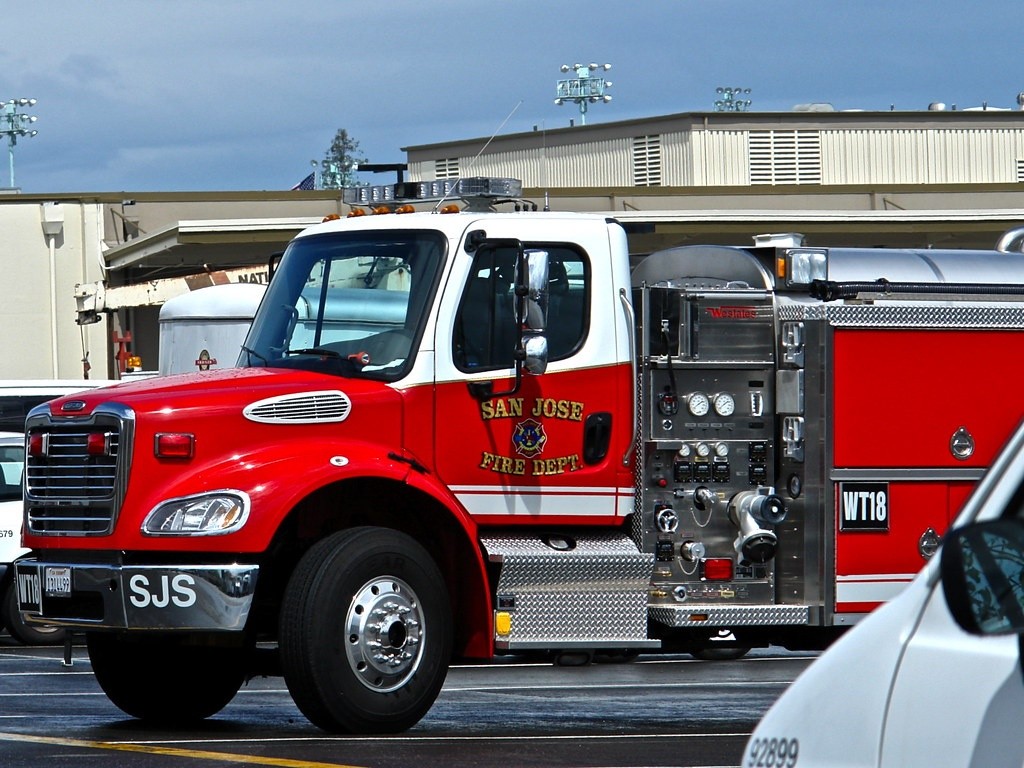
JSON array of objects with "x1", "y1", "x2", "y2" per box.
[{"x1": 11, "y1": 196, "x2": 1024, "y2": 736}]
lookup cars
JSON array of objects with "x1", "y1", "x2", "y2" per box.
[{"x1": 738, "y1": 417, "x2": 1024, "y2": 768}]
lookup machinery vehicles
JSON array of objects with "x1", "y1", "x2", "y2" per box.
[{"x1": 0, "y1": 246, "x2": 586, "y2": 645}]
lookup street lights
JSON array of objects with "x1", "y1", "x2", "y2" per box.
[
  {"x1": 554, "y1": 62, "x2": 613, "y2": 125},
  {"x1": 1, "y1": 96, "x2": 39, "y2": 190}
]
